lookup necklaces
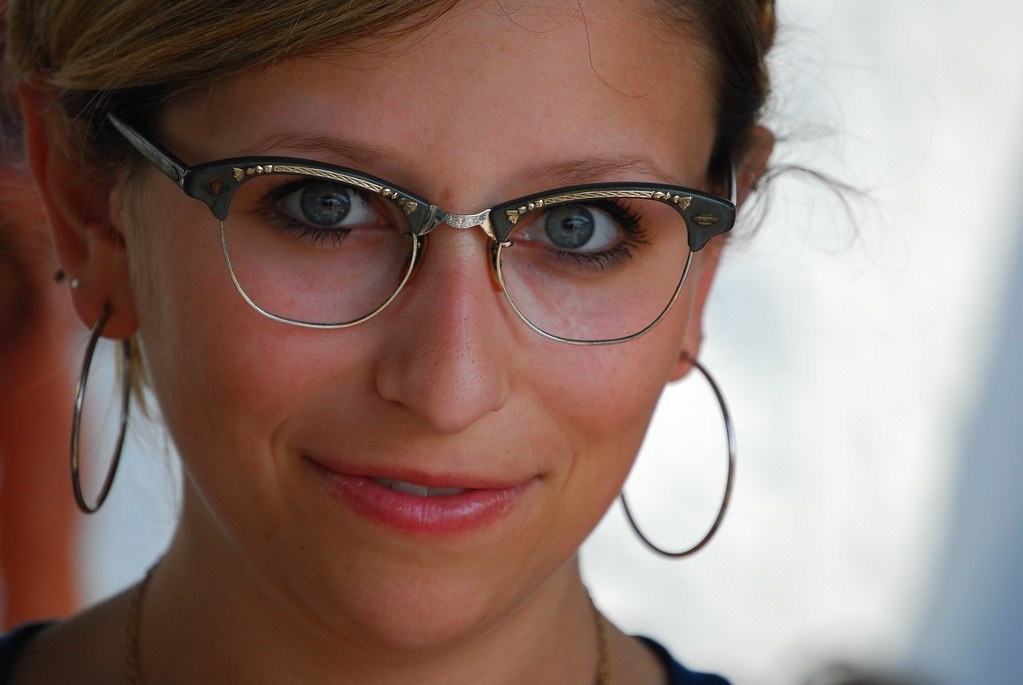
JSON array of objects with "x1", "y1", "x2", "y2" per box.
[{"x1": 124, "y1": 560, "x2": 610, "y2": 685}]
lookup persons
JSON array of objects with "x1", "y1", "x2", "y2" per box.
[{"x1": 0, "y1": 0, "x2": 778, "y2": 685}]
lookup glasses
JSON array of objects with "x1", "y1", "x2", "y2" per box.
[{"x1": 107, "y1": 109, "x2": 736, "y2": 344}]
